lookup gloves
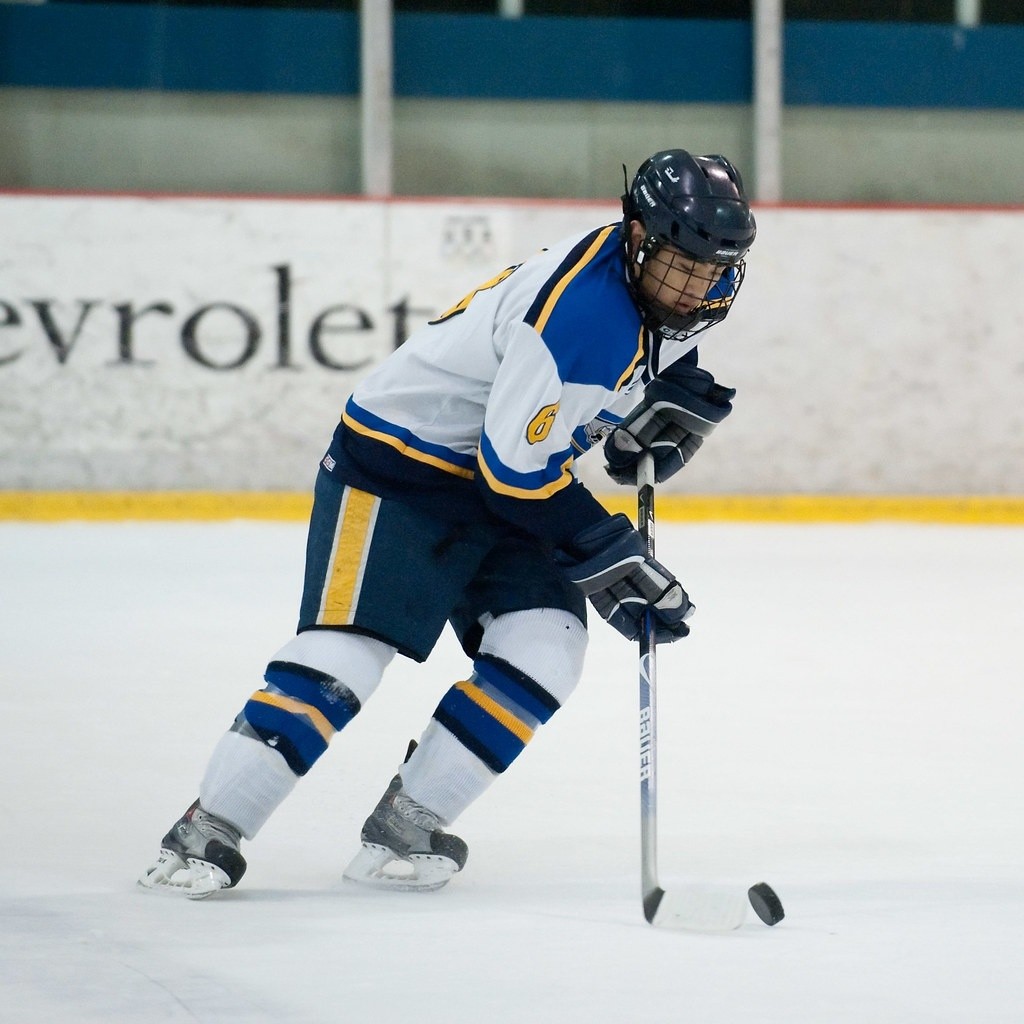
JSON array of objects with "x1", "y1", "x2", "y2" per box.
[
  {"x1": 553, "y1": 512, "x2": 696, "y2": 644},
  {"x1": 603, "y1": 363, "x2": 736, "y2": 486}
]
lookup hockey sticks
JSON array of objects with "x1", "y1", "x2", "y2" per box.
[{"x1": 637, "y1": 311, "x2": 742, "y2": 931}]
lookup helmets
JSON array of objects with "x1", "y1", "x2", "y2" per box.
[{"x1": 617, "y1": 148, "x2": 756, "y2": 340}]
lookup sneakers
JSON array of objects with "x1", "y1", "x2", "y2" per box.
[
  {"x1": 138, "y1": 796, "x2": 247, "y2": 900},
  {"x1": 343, "y1": 739, "x2": 469, "y2": 893}
]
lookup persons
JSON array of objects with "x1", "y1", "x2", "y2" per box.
[{"x1": 138, "y1": 148, "x2": 757, "y2": 899}]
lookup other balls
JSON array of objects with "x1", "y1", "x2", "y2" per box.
[{"x1": 749, "y1": 881, "x2": 786, "y2": 927}]
